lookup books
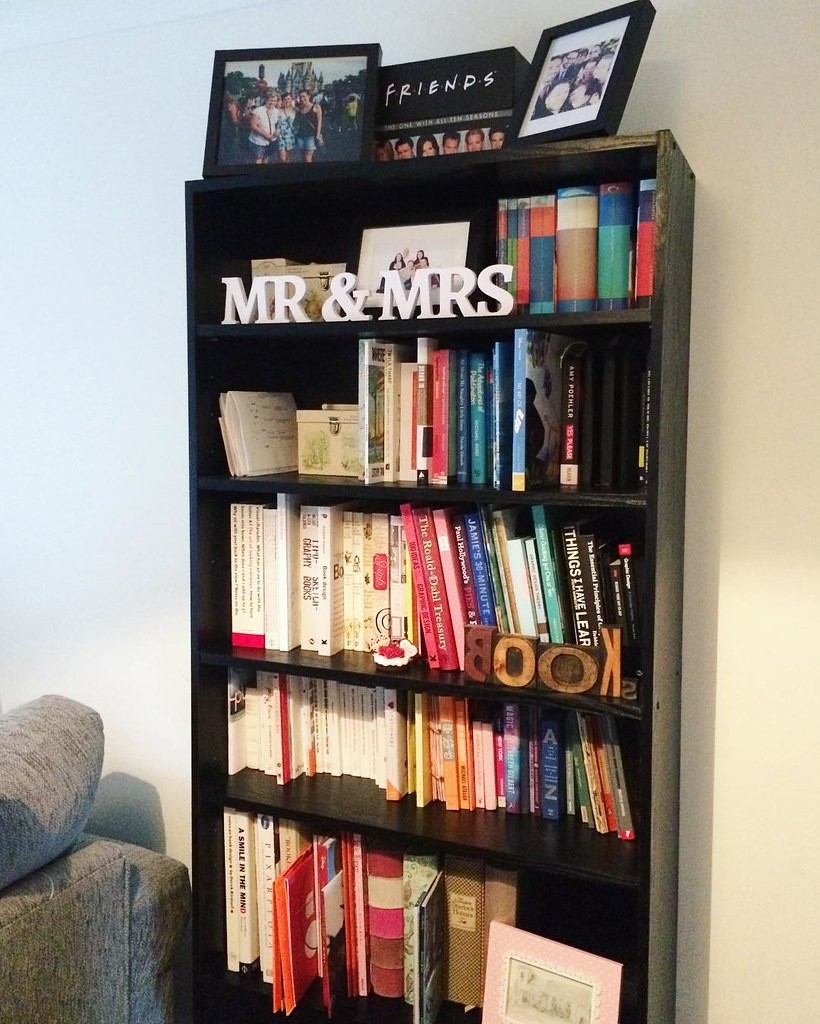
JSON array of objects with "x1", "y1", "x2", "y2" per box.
[
  {"x1": 494, "y1": 176, "x2": 656, "y2": 313},
  {"x1": 218, "y1": 327, "x2": 658, "y2": 1024}
]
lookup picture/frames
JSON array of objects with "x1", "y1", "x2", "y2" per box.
[
  {"x1": 482, "y1": 920, "x2": 623, "y2": 1024},
  {"x1": 355, "y1": 220, "x2": 470, "y2": 308},
  {"x1": 201, "y1": 42, "x2": 382, "y2": 179},
  {"x1": 501, "y1": 0, "x2": 656, "y2": 146}
]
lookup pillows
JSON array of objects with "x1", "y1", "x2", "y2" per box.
[{"x1": 0, "y1": 694, "x2": 105, "y2": 893}]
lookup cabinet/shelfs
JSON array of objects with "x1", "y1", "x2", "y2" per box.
[{"x1": 182, "y1": 129, "x2": 696, "y2": 1023}]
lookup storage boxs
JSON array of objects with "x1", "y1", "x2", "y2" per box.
[{"x1": 292, "y1": 408, "x2": 362, "y2": 476}]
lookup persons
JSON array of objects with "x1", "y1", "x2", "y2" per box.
[
  {"x1": 331, "y1": 88, "x2": 358, "y2": 134},
  {"x1": 465, "y1": 128, "x2": 485, "y2": 152},
  {"x1": 373, "y1": 139, "x2": 393, "y2": 162},
  {"x1": 249, "y1": 90, "x2": 323, "y2": 162},
  {"x1": 416, "y1": 134, "x2": 440, "y2": 157},
  {"x1": 376, "y1": 251, "x2": 440, "y2": 294},
  {"x1": 442, "y1": 131, "x2": 461, "y2": 155},
  {"x1": 395, "y1": 136, "x2": 416, "y2": 160},
  {"x1": 531, "y1": 37, "x2": 619, "y2": 121},
  {"x1": 488, "y1": 125, "x2": 507, "y2": 150}
]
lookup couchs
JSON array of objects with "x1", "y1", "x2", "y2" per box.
[{"x1": 0, "y1": 832, "x2": 191, "y2": 1024}]
